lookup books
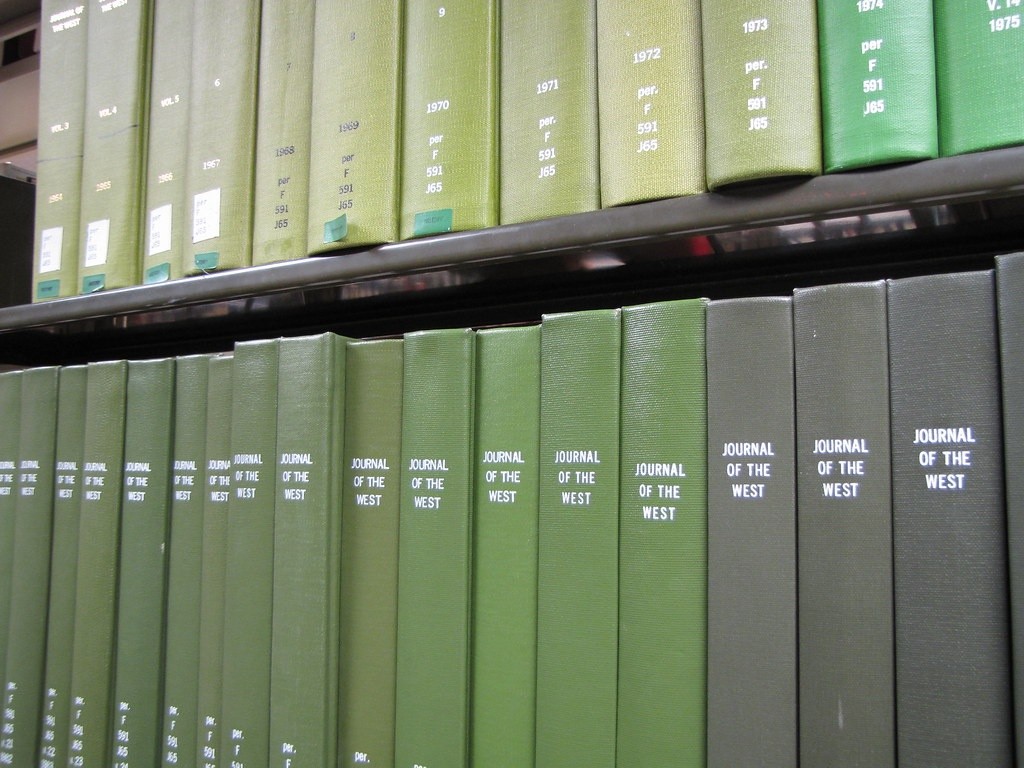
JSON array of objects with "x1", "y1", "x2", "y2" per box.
[
  {"x1": 0, "y1": 253, "x2": 1024, "y2": 768},
  {"x1": 33, "y1": 1, "x2": 1024, "y2": 308}
]
